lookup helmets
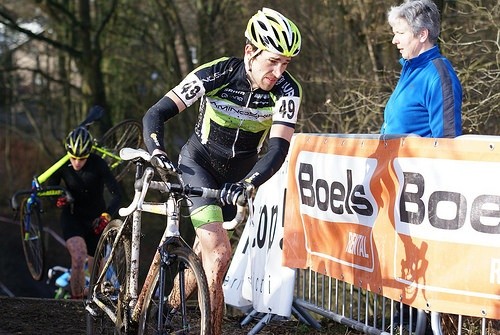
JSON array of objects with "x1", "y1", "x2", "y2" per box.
[
  {"x1": 245, "y1": 7, "x2": 301, "y2": 57},
  {"x1": 64, "y1": 127, "x2": 93, "y2": 159}
]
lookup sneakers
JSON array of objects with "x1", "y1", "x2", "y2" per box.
[{"x1": 150, "y1": 296, "x2": 176, "y2": 335}]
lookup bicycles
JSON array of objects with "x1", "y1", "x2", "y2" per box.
[
  {"x1": 11, "y1": 103, "x2": 144, "y2": 281},
  {"x1": 83, "y1": 146, "x2": 255, "y2": 335}
]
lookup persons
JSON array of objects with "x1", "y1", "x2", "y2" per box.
[
  {"x1": 142, "y1": 7, "x2": 303, "y2": 335},
  {"x1": 30, "y1": 127, "x2": 129, "y2": 297},
  {"x1": 381, "y1": 0, "x2": 464, "y2": 326}
]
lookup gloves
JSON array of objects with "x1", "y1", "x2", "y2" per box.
[
  {"x1": 152, "y1": 154, "x2": 183, "y2": 174},
  {"x1": 92, "y1": 213, "x2": 111, "y2": 235},
  {"x1": 57, "y1": 191, "x2": 73, "y2": 209},
  {"x1": 220, "y1": 181, "x2": 255, "y2": 205}
]
